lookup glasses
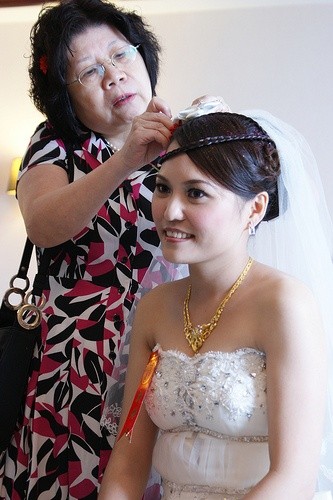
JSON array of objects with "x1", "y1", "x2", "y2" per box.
[{"x1": 65, "y1": 43, "x2": 141, "y2": 87}]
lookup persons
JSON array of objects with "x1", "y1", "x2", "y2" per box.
[
  {"x1": 0, "y1": 1, "x2": 234, "y2": 500},
  {"x1": 95, "y1": 100, "x2": 332, "y2": 499}
]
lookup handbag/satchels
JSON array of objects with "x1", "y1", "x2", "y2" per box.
[{"x1": 0, "y1": 283, "x2": 41, "y2": 453}]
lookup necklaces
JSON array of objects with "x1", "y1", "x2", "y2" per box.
[
  {"x1": 104, "y1": 137, "x2": 122, "y2": 152},
  {"x1": 182, "y1": 254, "x2": 254, "y2": 354}
]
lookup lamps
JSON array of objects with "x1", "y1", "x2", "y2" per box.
[{"x1": 6, "y1": 157, "x2": 23, "y2": 196}]
